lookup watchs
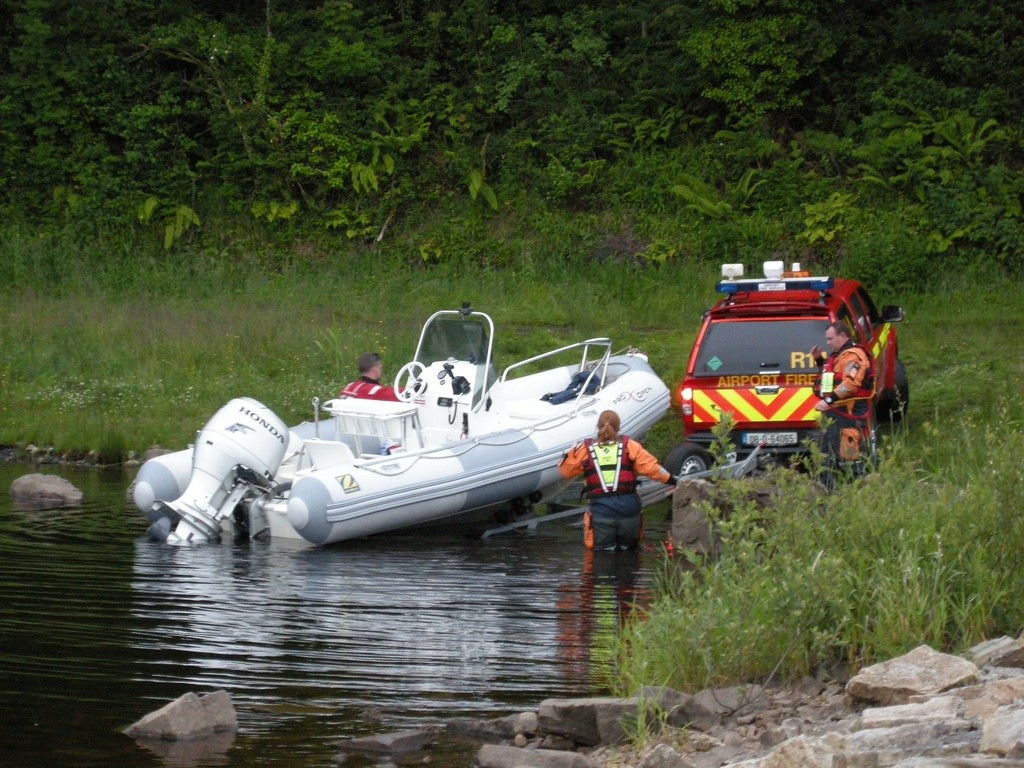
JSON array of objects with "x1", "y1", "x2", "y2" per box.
[{"x1": 825, "y1": 397, "x2": 833, "y2": 405}]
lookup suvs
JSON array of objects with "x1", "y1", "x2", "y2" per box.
[{"x1": 673, "y1": 261, "x2": 910, "y2": 479}]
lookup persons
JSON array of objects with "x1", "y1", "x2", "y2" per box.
[
  {"x1": 559, "y1": 410, "x2": 679, "y2": 583},
  {"x1": 811, "y1": 321, "x2": 877, "y2": 488},
  {"x1": 339, "y1": 353, "x2": 404, "y2": 402}
]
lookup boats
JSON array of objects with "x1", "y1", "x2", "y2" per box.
[{"x1": 132, "y1": 307, "x2": 671, "y2": 550}]
